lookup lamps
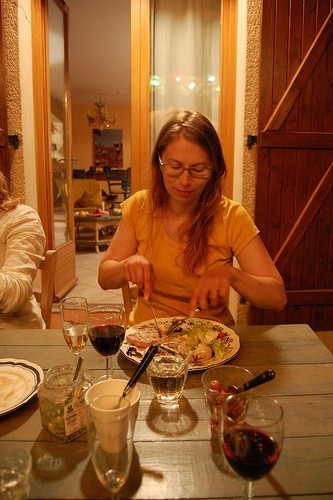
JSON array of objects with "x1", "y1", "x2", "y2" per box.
[{"x1": 86, "y1": 94, "x2": 118, "y2": 135}]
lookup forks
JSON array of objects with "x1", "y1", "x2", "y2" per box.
[{"x1": 166, "y1": 306, "x2": 200, "y2": 336}]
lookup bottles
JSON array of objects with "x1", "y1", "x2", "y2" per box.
[{"x1": 37, "y1": 364, "x2": 92, "y2": 442}]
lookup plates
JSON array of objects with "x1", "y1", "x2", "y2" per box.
[
  {"x1": 120, "y1": 317, "x2": 240, "y2": 371},
  {"x1": 0, "y1": 357, "x2": 45, "y2": 417}
]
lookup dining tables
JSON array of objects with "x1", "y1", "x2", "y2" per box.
[{"x1": 0, "y1": 324, "x2": 333, "y2": 500}]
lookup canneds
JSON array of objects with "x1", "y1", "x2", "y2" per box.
[{"x1": 39, "y1": 364, "x2": 94, "y2": 442}]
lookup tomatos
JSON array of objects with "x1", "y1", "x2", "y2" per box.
[{"x1": 209, "y1": 380, "x2": 232, "y2": 405}]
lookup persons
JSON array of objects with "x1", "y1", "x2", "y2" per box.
[
  {"x1": 0, "y1": 172, "x2": 48, "y2": 331},
  {"x1": 98, "y1": 107, "x2": 286, "y2": 328}
]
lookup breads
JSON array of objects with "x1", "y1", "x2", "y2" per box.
[{"x1": 176, "y1": 340, "x2": 212, "y2": 363}]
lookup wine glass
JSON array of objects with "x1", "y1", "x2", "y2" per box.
[
  {"x1": 220, "y1": 392, "x2": 285, "y2": 500},
  {"x1": 60, "y1": 296, "x2": 92, "y2": 388},
  {"x1": 85, "y1": 395, "x2": 134, "y2": 500},
  {"x1": 87, "y1": 304, "x2": 127, "y2": 386}
]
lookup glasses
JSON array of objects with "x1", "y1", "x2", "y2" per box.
[{"x1": 158, "y1": 154, "x2": 213, "y2": 179}]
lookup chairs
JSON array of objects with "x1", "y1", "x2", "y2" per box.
[{"x1": 34, "y1": 250, "x2": 57, "y2": 330}]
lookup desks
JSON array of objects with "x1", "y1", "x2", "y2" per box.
[{"x1": 73, "y1": 213, "x2": 121, "y2": 254}]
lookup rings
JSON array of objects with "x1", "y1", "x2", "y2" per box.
[{"x1": 208, "y1": 289, "x2": 220, "y2": 294}]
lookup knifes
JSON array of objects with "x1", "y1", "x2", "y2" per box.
[{"x1": 142, "y1": 289, "x2": 162, "y2": 339}]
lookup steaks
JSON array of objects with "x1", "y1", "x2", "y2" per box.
[{"x1": 126, "y1": 327, "x2": 172, "y2": 347}]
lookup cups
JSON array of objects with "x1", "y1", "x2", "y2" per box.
[
  {"x1": 0, "y1": 442, "x2": 32, "y2": 500},
  {"x1": 85, "y1": 380, "x2": 141, "y2": 434},
  {"x1": 202, "y1": 365, "x2": 256, "y2": 433},
  {"x1": 145, "y1": 342, "x2": 191, "y2": 404}
]
